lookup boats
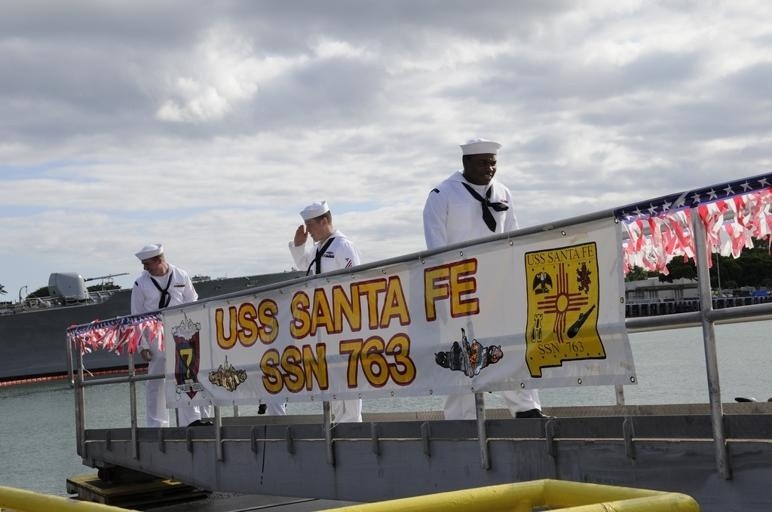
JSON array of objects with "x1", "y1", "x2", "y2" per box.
[
  {"x1": 1, "y1": 167, "x2": 770, "y2": 512},
  {"x1": 0, "y1": 263, "x2": 315, "y2": 391}
]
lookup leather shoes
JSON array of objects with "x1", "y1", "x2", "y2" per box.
[
  {"x1": 515, "y1": 409, "x2": 557, "y2": 418},
  {"x1": 187, "y1": 420, "x2": 213, "y2": 427}
]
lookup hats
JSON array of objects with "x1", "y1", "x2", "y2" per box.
[
  {"x1": 134, "y1": 243, "x2": 165, "y2": 260},
  {"x1": 459, "y1": 138, "x2": 503, "y2": 156},
  {"x1": 298, "y1": 200, "x2": 330, "y2": 222}
]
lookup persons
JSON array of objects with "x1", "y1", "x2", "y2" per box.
[
  {"x1": 131, "y1": 242, "x2": 210, "y2": 429},
  {"x1": 288, "y1": 199, "x2": 363, "y2": 423},
  {"x1": 424, "y1": 138, "x2": 556, "y2": 418}
]
